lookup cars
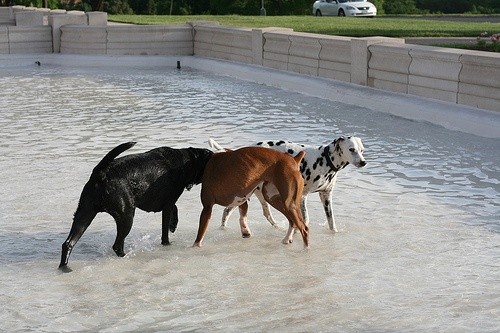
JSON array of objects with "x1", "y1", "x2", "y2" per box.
[{"x1": 312, "y1": 0, "x2": 377, "y2": 17}]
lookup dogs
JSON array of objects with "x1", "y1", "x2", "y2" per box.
[
  {"x1": 57, "y1": 142, "x2": 213, "y2": 273},
  {"x1": 207, "y1": 135, "x2": 368, "y2": 233},
  {"x1": 192, "y1": 147, "x2": 310, "y2": 249}
]
